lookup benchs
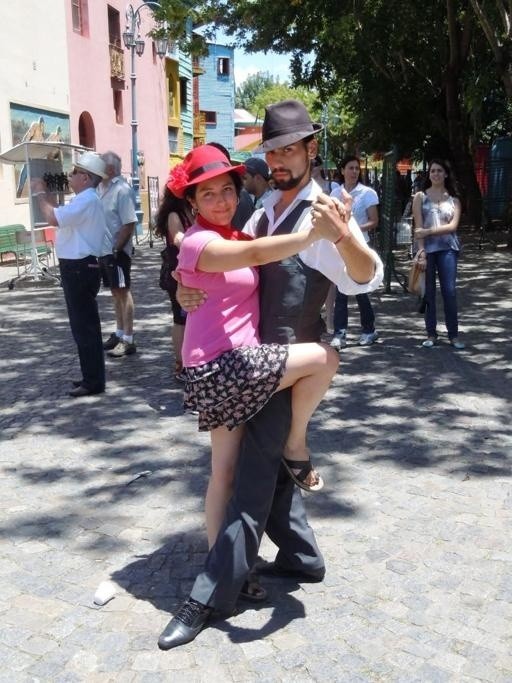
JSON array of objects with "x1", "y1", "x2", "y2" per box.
[{"x1": 0, "y1": 224, "x2": 52, "y2": 266}]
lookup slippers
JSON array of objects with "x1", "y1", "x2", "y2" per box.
[
  {"x1": 240, "y1": 581, "x2": 267, "y2": 601},
  {"x1": 280, "y1": 453, "x2": 325, "y2": 493}
]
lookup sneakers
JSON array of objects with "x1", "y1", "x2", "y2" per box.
[
  {"x1": 103, "y1": 332, "x2": 122, "y2": 350},
  {"x1": 330, "y1": 330, "x2": 347, "y2": 351},
  {"x1": 422, "y1": 337, "x2": 437, "y2": 348},
  {"x1": 358, "y1": 329, "x2": 378, "y2": 346},
  {"x1": 175, "y1": 371, "x2": 186, "y2": 383},
  {"x1": 449, "y1": 341, "x2": 466, "y2": 349},
  {"x1": 106, "y1": 339, "x2": 137, "y2": 357}
]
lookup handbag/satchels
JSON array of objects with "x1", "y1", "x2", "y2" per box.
[{"x1": 406, "y1": 249, "x2": 427, "y2": 299}]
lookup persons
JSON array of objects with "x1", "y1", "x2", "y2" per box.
[
  {"x1": 411, "y1": 158, "x2": 464, "y2": 349},
  {"x1": 232, "y1": 157, "x2": 276, "y2": 231},
  {"x1": 46, "y1": 126, "x2": 64, "y2": 168},
  {"x1": 60, "y1": 172, "x2": 69, "y2": 191},
  {"x1": 42, "y1": 172, "x2": 53, "y2": 192},
  {"x1": 29, "y1": 151, "x2": 110, "y2": 398},
  {"x1": 329, "y1": 155, "x2": 380, "y2": 351},
  {"x1": 310, "y1": 155, "x2": 341, "y2": 195},
  {"x1": 157, "y1": 100, "x2": 385, "y2": 651},
  {"x1": 97, "y1": 151, "x2": 139, "y2": 357},
  {"x1": 48, "y1": 172, "x2": 56, "y2": 192},
  {"x1": 163, "y1": 143, "x2": 347, "y2": 601},
  {"x1": 157, "y1": 181, "x2": 195, "y2": 374},
  {"x1": 16, "y1": 117, "x2": 45, "y2": 198},
  {"x1": 54, "y1": 172, "x2": 63, "y2": 191}
]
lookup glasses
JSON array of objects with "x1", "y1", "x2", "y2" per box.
[{"x1": 72, "y1": 167, "x2": 93, "y2": 178}]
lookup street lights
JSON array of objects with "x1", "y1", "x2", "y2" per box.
[{"x1": 123, "y1": 2, "x2": 168, "y2": 235}]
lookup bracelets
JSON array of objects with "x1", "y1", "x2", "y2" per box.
[
  {"x1": 333, "y1": 231, "x2": 351, "y2": 244},
  {"x1": 112, "y1": 247, "x2": 121, "y2": 253},
  {"x1": 32, "y1": 190, "x2": 46, "y2": 198}
]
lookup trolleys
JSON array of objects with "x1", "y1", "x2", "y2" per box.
[{"x1": 393, "y1": 216, "x2": 414, "y2": 260}]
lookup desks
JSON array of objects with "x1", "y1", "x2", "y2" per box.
[{"x1": 9, "y1": 227, "x2": 62, "y2": 290}]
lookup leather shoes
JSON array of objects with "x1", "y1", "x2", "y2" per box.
[
  {"x1": 69, "y1": 386, "x2": 105, "y2": 397},
  {"x1": 254, "y1": 558, "x2": 326, "y2": 583},
  {"x1": 72, "y1": 378, "x2": 85, "y2": 386},
  {"x1": 157, "y1": 596, "x2": 216, "y2": 650}
]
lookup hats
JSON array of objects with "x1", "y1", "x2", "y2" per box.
[
  {"x1": 166, "y1": 144, "x2": 248, "y2": 200},
  {"x1": 250, "y1": 101, "x2": 325, "y2": 155},
  {"x1": 71, "y1": 151, "x2": 111, "y2": 180},
  {"x1": 243, "y1": 157, "x2": 270, "y2": 180}
]
